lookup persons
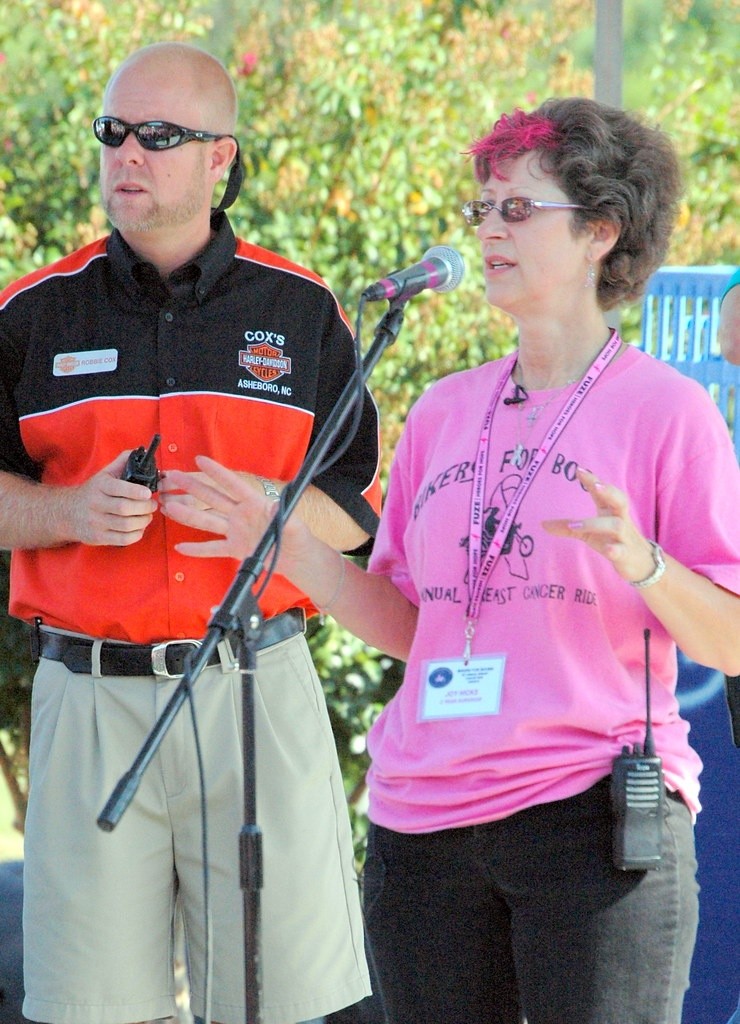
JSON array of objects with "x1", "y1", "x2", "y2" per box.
[
  {"x1": 167, "y1": 96, "x2": 740, "y2": 1024},
  {"x1": 0, "y1": 44, "x2": 381, "y2": 1024}
]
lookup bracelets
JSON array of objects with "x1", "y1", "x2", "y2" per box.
[
  {"x1": 311, "y1": 554, "x2": 346, "y2": 625},
  {"x1": 257, "y1": 475, "x2": 279, "y2": 497}
]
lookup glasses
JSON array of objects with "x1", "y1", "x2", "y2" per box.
[
  {"x1": 92, "y1": 116, "x2": 232, "y2": 152},
  {"x1": 462, "y1": 197, "x2": 600, "y2": 226}
]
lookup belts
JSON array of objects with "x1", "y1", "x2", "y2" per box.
[{"x1": 39, "y1": 604, "x2": 304, "y2": 677}]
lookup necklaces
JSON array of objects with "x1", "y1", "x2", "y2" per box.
[{"x1": 511, "y1": 363, "x2": 592, "y2": 466}]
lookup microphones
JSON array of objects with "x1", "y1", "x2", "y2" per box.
[
  {"x1": 504, "y1": 397, "x2": 526, "y2": 405},
  {"x1": 361, "y1": 245, "x2": 464, "y2": 302}
]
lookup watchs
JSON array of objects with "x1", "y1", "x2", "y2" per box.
[{"x1": 630, "y1": 539, "x2": 667, "y2": 589}]
